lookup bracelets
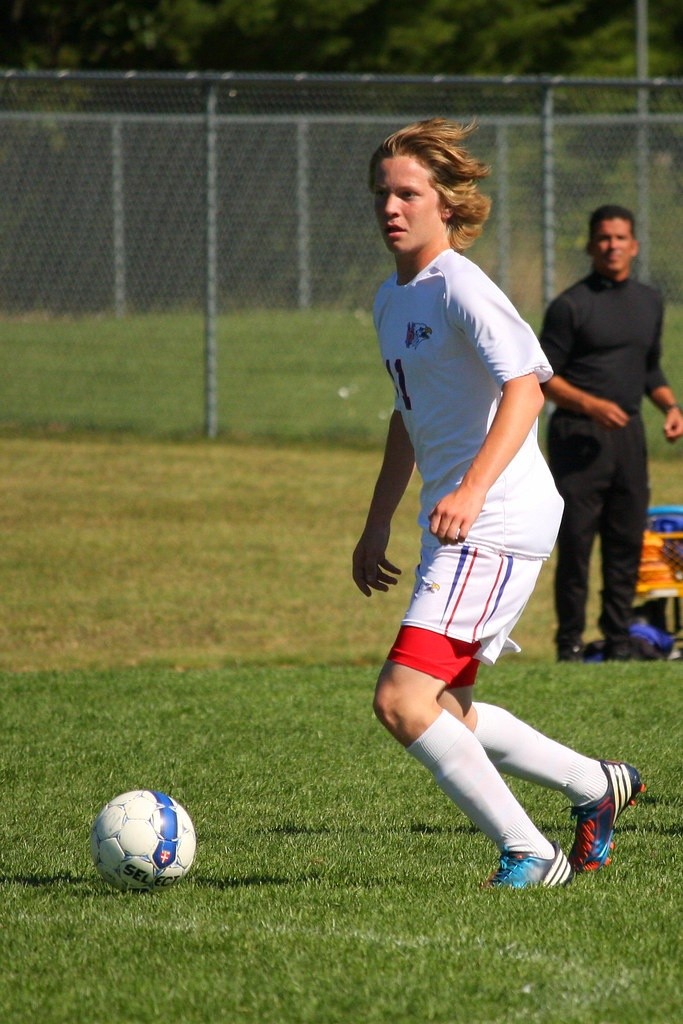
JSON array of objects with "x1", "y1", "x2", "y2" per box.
[{"x1": 664, "y1": 404, "x2": 682, "y2": 415}]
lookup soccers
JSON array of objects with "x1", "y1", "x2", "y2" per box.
[{"x1": 87, "y1": 787, "x2": 197, "y2": 891}]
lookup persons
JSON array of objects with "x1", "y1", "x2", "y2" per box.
[
  {"x1": 538, "y1": 203, "x2": 683, "y2": 663},
  {"x1": 350, "y1": 118, "x2": 644, "y2": 894}
]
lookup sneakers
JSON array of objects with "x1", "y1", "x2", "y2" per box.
[
  {"x1": 569, "y1": 760, "x2": 647, "y2": 872},
  {"x1": 483, "y1": 839, "x2": 573, "y2": 892}
]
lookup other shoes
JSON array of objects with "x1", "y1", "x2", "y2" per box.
[
  {"x1": 602, "y1": 642, "x2": 631, "y2": 660},
  {"x1": 556, "y1": 638, "x2": 585, "y2": 662}
]
